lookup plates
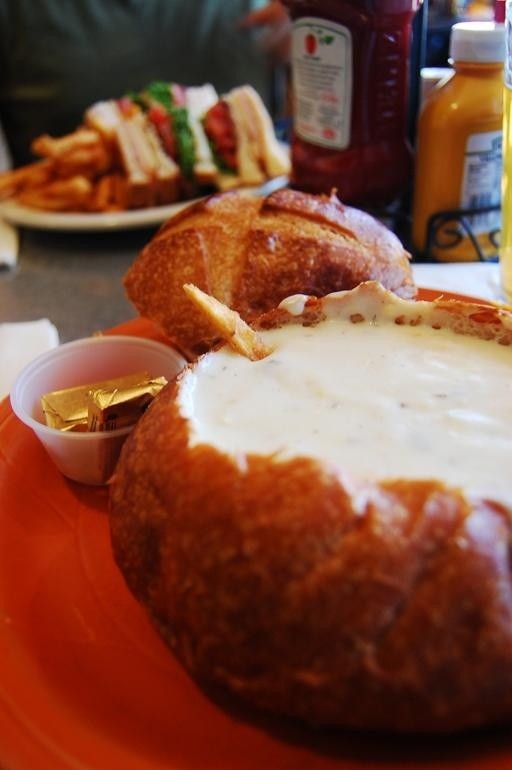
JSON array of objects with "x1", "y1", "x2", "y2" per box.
[
  {"x1": 1, "y1": 288, "x2": 512, "y2": 770},
  {"x1": 0, "y1": 142, "x2": 292, "y2": 232}
]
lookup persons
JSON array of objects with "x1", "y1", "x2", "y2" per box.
[{"x1": 1, "y1": 1, "x2": 296, "y2": 171}]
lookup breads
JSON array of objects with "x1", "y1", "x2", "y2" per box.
[{"x1": 125, "y1": 187, "x2": 418, "y2": 358}]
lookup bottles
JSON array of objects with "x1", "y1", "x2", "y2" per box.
[
  {"x1": 414, "y1": 19, "x2": 511, "y2": 261},
  {"x1": 282, "y1": 0, "x2": 416, "y2": 202}
]
